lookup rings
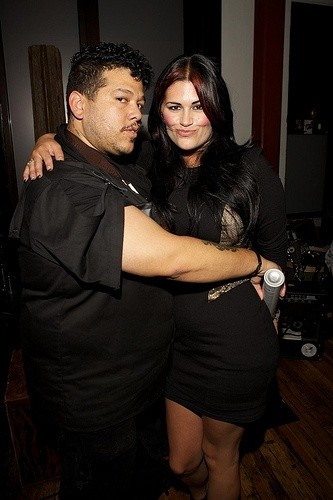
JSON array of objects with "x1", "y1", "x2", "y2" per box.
[{"x1": 26, "y1": 160, "x2": 35, "y2": 166}]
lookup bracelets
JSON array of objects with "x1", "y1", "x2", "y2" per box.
[{"x1": 247, "y1": 248, "x2": 262, "y2": 279}]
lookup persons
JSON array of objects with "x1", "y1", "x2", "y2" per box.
[
  {"x1": 0, "y1": 42, "x2": 286, "y2": 500},
  {"x1": 22, "y1": 55, "x2": 288, "y2": 500}
]
